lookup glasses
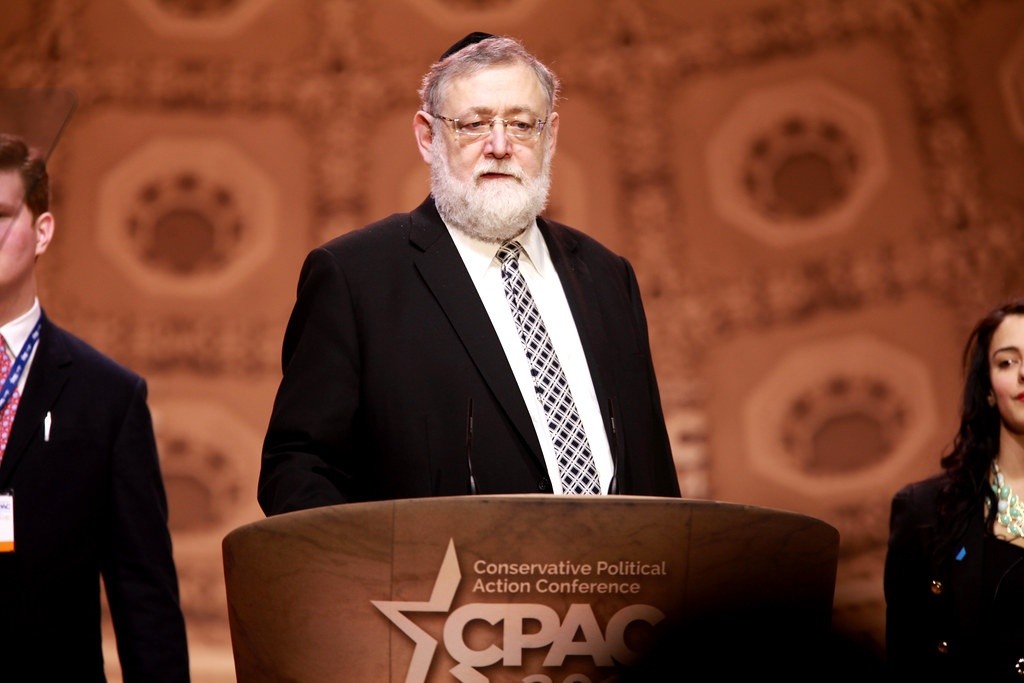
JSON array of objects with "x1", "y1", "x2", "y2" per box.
[{"x1": 438, "y1": 115, "x2": 546, "y2": 138}]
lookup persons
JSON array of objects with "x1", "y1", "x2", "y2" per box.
[
  {"x1": 882, "y1": 299, "x2": 1024, "y2": 683},
  {"x1": 0, "y1": 133, "x2": 189, "y2": 683},
  {"x1": 257, "y1": 31, "x2": 683, "y2": 517}
]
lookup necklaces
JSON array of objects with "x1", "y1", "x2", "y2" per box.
[{"x1": 986, "y1": 457, "x2": 1024, "y2": 538}]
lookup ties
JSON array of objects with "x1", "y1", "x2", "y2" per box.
[
  {"x1": 496, "y1": 244, "x2": 600, "y2": 496},
  {"x1": 0, "y1": 335, "x2": 19, "y2": 462}
]
